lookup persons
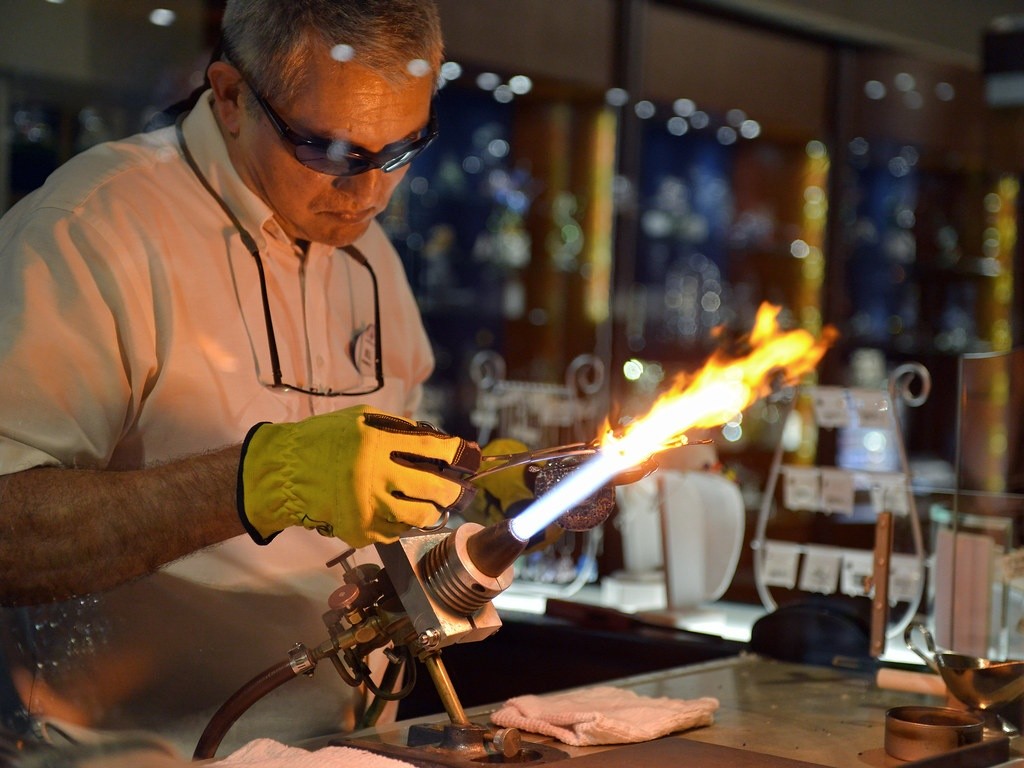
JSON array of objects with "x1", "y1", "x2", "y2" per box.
[{"x1": 2, "y1": 1, "x2": 658, "y2": 768}]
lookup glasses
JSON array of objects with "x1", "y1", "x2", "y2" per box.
[
  {"x1": 224, "y1": 46, "x2": 439, "y2": 176},
  {"x1": 240, "y1": 230, "x2": 384, "y2": 398}
]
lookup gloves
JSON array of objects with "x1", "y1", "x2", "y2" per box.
[
  {"x1": 458, "y1": 438, "x2": 566, "y2": 553},
  {"x1": 235, "y1": 405, "x2": 482, "y2": 548}
]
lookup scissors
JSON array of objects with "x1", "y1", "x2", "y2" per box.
[{"x1": 415, "y1": 421, "x2": 596, "y2": 534}]
